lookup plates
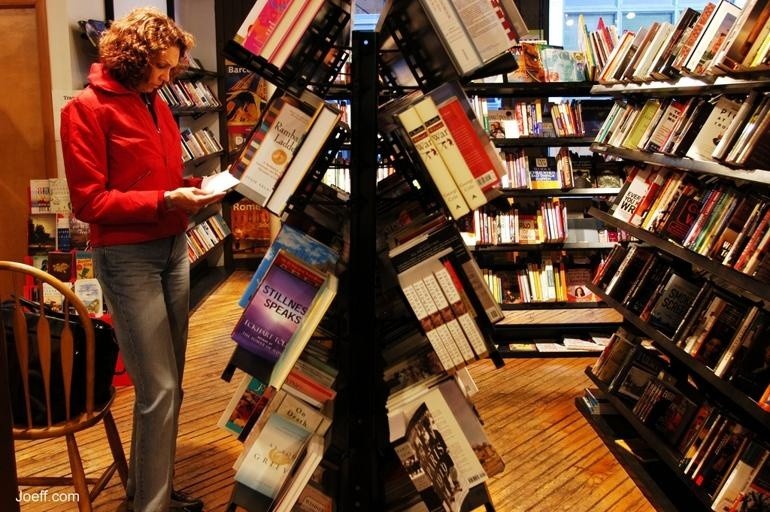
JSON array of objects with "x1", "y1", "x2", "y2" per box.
[
  {"x1": 220, "y1": 1, "x2": 360, "y2": 511},
  {"x1": 461, "y1": 81, "x2": 619, "y2": 357},
  {"x1": 575, "y1": 69, "x2": 769, "y2": 511},
  {"x1": 77, "y1": 18, "x2": 233, "y2": 320},
  {"x1": 368, "y1": 0, "x2": 519, "y2": 512}
]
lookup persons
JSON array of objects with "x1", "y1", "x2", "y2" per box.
[{"x1": 59, "y1": 7, "x2": 237, "y2": 512}]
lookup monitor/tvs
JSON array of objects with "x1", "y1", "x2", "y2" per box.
[
  {"x1": 418, "y1": 0, "x2": 530, "y2": 75},
  {"x1": 459, "y1": 197, "x2": 639, "y2": 247},
  {"x1": 29, "y1": 177, "x2": 104, "y2": 319},
  {"x1": 156, "y1": 79, "x2": 223, "y2": 113},
  {"x1": 377, "y1": 298, "x2": 505, "y2": 512},
  {"x1": 576, "y1": 13, "x2": 619, "y2": 81},
  {"x1": 607, "y1": 164, "x2": 770, "y2": 283},
  {"x1": 466, "y1": 93, "x2": 611, "y2": 140},
  {"x1": 231, "y1": 0, "x2": 327, "y2": 69},
  {"x1": 591, "y1": 323, "x2": 770, "y2": 512},
  {"x1": 507, "y1": 327, "x2": 616, "y2": 355},
  {"x1": 581, "y1": 385, "x2": 658, "y2": 464},
  {"x1": 596, "y1": 0, "x2": 770, "y2": 83},
  {"x1": 217, "y1": 319, "x2": 339, "y2": 512},
  {"x1": 183, "y1": 50, "x2": 204, "y2": 72},
  {"x1": 591, "y1": 243, "x2": 770, "y2": 416},
  {"x1": 230, "y1": 196, "x2": 346, "y2": 392},
  {"x1": 377, "y1": 173, "x2": 506, "y2": 377},
  {"x1": 592, "y1": 86, "x2": 770, "y2": 167},
  {"x1": 179, "y1": 126, "x2": 224, "y2": 164},
  {"x1": 227, "y1": 96, "x2": 344, "y2": 216},
  {"x1": 185, "y1": 212, "x2": 231, "y2": 266},
  {"x1": 494, "y1": 144, "x2": 632, "y2": 193},
  {"x1": 472, "y1": 251, "x2": 608, "y2": 306},
  {"x1": 470, "y1": 28, "x2": 588, "y2": 84},
  {"x1": 377, "y1": 79, "x2": 507, "y2": 222},
  {"x1": 79, "y1": 19, "x2": 110, "y2": 49}
]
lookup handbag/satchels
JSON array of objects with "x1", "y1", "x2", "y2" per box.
[{"x1": 163, "y1": 190, "x2": 173, "y2": 209}]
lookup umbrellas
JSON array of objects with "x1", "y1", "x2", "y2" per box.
[{"x1": 127, "y1": 491, "x2": 204, "y2": 512}]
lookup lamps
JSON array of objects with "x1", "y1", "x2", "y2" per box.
[{"x1": 0, "y1": 260, "x2": 128, "y2": 511}]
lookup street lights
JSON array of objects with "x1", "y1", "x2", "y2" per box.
[{"x1": 0, "y1": 293, "x2": 119, "y2": 427}]
